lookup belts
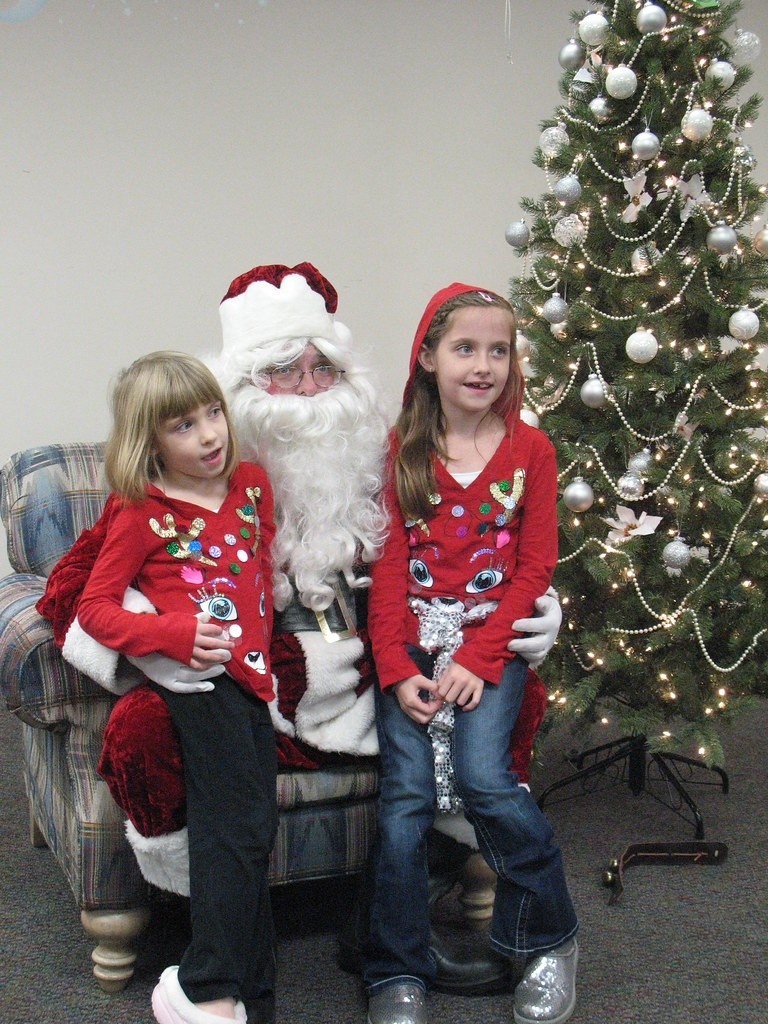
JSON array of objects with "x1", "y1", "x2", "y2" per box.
[{"x1": 271, "y1": 564, "x2": 371, "y2": 644}]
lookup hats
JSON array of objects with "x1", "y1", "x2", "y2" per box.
[{"x1": 219, "y1": 262, "x2": 338, "y2": 358}]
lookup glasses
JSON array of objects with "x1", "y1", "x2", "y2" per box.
[{"x1": 266, "y1": 366, "x2": 346, "y2": 390}]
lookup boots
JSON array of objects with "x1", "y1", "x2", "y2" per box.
[
  {"x1": 140, "y1": 884, "x2": 193, "y2": 980},
  {"x1": 338, "y1": 821, "x2": 515, "y2": 995}
]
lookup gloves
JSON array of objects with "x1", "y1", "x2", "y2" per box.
[
  {"x1": 122, "y1": 612, "x2": 230, "y2": 694},
  {"x1": 508, "y1": 595, "x2": 562, "y2": 663}
]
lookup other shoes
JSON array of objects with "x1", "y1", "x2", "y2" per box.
[
  {"x1": 513, "y1": 936, "x2": 578, "y2": 1024},
  {"x1": 151, "y1": 964, "x2": 248, "y2": 1024},
  {"x1": 367, "y1": 986, "x2": 428, "y2": 1024}
]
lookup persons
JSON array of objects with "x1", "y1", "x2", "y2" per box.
[
  {"x1": 362, "y1": 282, "x2": 579, "y2": 1024},
  {"x1": 33, "y1": 257, "x2": 564, "y2": 1024},
  {"x1": 75, "y1": 350, "x2": 276, "y2": 1024}
]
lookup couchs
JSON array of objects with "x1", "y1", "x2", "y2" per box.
[{"x1": 0, "y1": 437, "x2": 494, "y2": 994}]
